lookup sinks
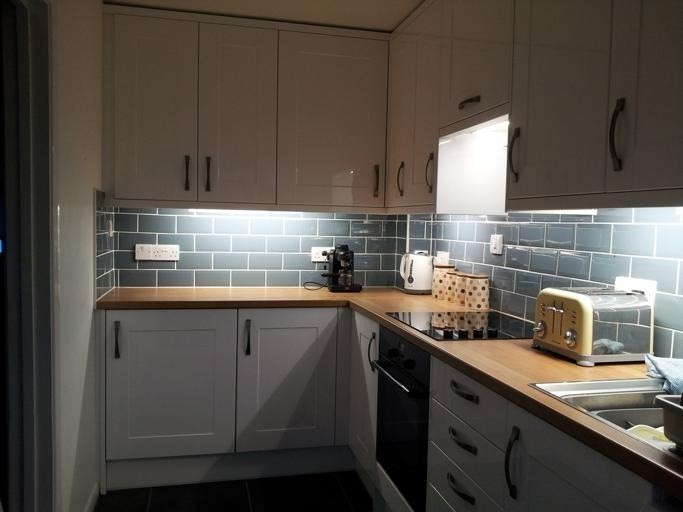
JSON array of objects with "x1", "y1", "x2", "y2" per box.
[{"x1": 528, "y1": 379, "x2": 674, "y2": 455}]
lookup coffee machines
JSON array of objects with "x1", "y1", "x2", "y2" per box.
[{"x1": 320, "y1": 243, "x2": 364, "y2": 293}]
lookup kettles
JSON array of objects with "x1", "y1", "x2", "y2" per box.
[{"x1": 397, "y1": 248, "x2": 436, "y2": 296}]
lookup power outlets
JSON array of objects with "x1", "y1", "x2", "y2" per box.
[{"x1": 150, "y1": 245, "x2": 180, "y2": 262}]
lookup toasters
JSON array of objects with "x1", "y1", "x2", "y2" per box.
[{"x1": 529, "y1": 284, "x2": 655, "y2": 369}]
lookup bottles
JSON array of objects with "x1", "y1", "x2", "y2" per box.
[
  {"x1": 431, "y1": 263, "x2": 456, "y2": 302},
  {"x1": 451, "y1": 272, "x2": 466, "y2": 305},
  {"x1": 464, "y1": 272, "x2": 491, "y2": 311},
  {"x1": 442, "y1": 270, "x2": 456, "y2": 304}
]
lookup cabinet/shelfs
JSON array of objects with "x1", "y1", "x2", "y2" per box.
[
  {"x1": 276, "y1": 30, "x2": 384, "y2": 210},
  {"x1": 508, "y1": 0, "x2": 681, "y2": 201},
  {"x1": 106, "y1": 307, "x2": 235, "y2": 461},
  {"x1": 108, "y1": 12, "x2": 275, "y2": 206},
  {"x1": 348, "y1": 314, "x2": 378, "y2": 481},
  {"x1": 425, "y1": 358, "x2": 653, "y2": 512},
  {"x1": 382, "y1": 0, "x2": 513, "y2": 216},
  {"x1": 235, "y1": 307, "x2": 337, "y2": 454}
]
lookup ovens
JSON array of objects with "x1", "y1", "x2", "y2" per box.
[{"x1": 374, "y1": 319, "x2": 432, "y2": 512}]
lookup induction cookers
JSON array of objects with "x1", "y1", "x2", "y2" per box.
[{"x1": 384, "y1": 311, "x2": 536, "y2": 346}]
